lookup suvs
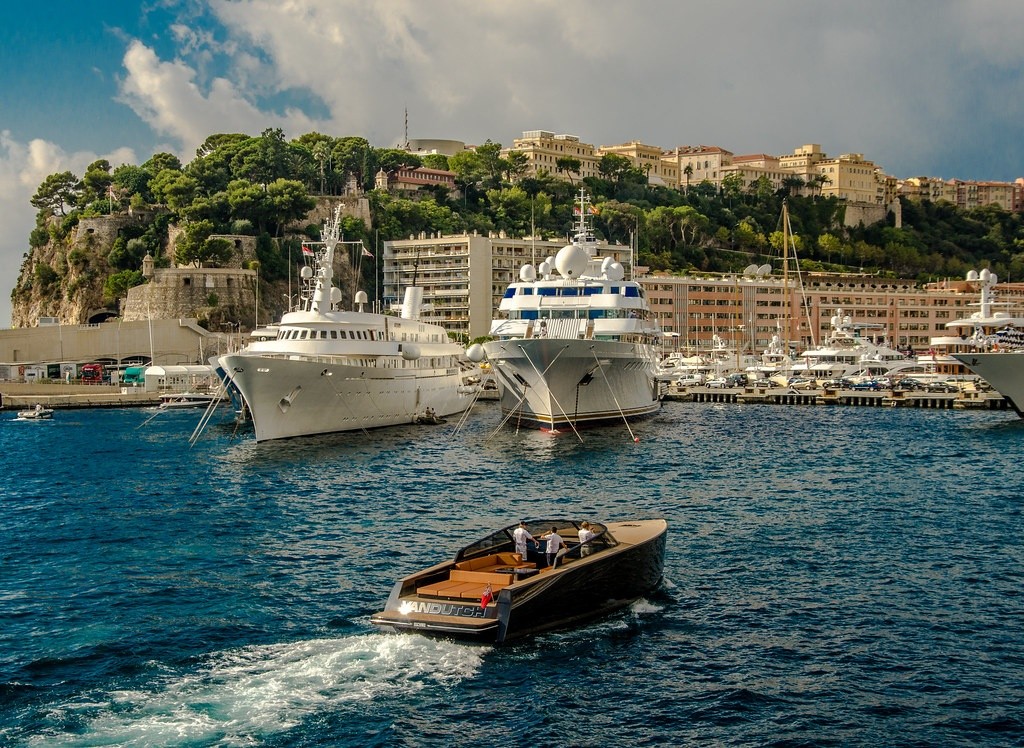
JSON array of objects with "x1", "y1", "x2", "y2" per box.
[
  {"x1": 705, "y1": 377, "x2": 734, "y2": 389},
  {"x1": 789, "y1": 377, "x2": 797, "y2": 384}
]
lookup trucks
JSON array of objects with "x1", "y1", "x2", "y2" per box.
[{"x1": 81, "y1": 364, "x2": 149, "y2": 387}]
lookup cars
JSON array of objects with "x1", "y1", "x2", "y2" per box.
[
  {"x1": 877, "y1": 378, "x2": 893, "y2": 389},
  {"x1": 921, "y1": 380, "x2": 954, "y2": 393},
  {"x1": 751, "y1": 378, "x2": 778, "y2": 387},
  {"x1": 975, "y1": 380, "x2": 991, "y2": 391},
  {"x1": 822, "y1": 377, "x2": 853, "y2": 387},
  {"x1": 849, "y1": 380, "x2": 881, "y2": 392},
  {"x1": 896, "y1": 378, "x2": 926, "y2": 390}
]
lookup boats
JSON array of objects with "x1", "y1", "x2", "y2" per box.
[
  {"x1": 481, "y1": 185, "x2": 661, "y2": 431},
  {"x1": 658, "y1": 193, "x2": 1024, "y2": 419},
  {"x1": 209, "y1": 204, "x2": 499, "y2": 444},
  {"x1": 369, "y1": 518, "x2": 668, "y2": 642},
  {"x1": 17, "y1": 404, "x2": 54, "y2": 419}
]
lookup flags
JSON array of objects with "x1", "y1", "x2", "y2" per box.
[
  {"x1": 481, "y1": 585, "x2": 492, "y2": 609},
  {"x1": 573, "y1": 205, "x2": 600, "y2": 215},
  {"x1": 302, "y1": 246, "x2": 315, "y2": 256},
  {"x1": 362, "y1": 245, "x2": 374, "y2": 258}
]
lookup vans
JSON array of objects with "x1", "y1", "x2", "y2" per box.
[
  {"x1": 787, "y1": 379, "x2": 817, "y2": 390},
  {"x1": 729, "y1": 373, "x2": 748, "y2": 386},
  {"x1": 676, "y1": 374, "x2": 704, "y2": 387}
]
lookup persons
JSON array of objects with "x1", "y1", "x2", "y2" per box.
[
  {"x1": 578, "y1": 521, "x2": 597, "y2": 557},
  {"x1": 971, "y1": 336, "x2": 998, "y2": 353},
  {"x1": 426, "y1": 407, "x2": 438, "y2": 419},
  {"x1": 513, "y1": 521, "x2": 539, "y2": 562},
  {"x1": 541, "y1": 527, "x2": 567, "y2": 566}
]
